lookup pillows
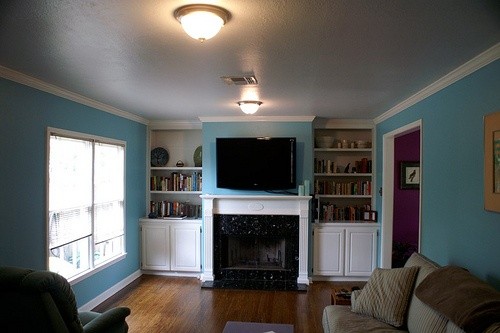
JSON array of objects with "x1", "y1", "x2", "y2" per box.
[{"x1": 350, "y1": 264, "x2": 421, "y2": 329}]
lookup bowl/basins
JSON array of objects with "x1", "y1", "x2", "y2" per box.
[
  {"x1": 356, "y1": 139, "x2": 369, "y2": 148},
  {"x1": 316, "y1": 136, "x2": 336, "y2": 148}
]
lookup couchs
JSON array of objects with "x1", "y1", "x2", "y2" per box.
[
  {"x1": 323, "y1": 250, "x2": 499, "y2": 333},
  {"x1": 1, "y1": 265, "x2": 131, "y2": 332}
]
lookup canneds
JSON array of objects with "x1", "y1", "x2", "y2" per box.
[{"x1": 349, "y1": 140, "x2": 355, "y2": 148}]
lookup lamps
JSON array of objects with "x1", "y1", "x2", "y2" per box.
[
  {"x1": 235, "y1": 99, "x2": 264, "y2": 115},
  {"x1": 173, "y1": 3, "x2": 232, "y2": 43}
]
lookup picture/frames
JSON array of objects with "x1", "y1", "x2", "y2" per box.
[
  {"x1": 397, "y1": 160, "x2": 420, "y2": 190},
  {"x1": 483, "y1": 111, "x2": 500, "y2": 214}
]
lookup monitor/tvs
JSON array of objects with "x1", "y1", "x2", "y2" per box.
[{"x1": 215, "y1": 137, "x2": 297, "y2": 190}]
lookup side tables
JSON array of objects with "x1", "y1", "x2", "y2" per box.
[{"x1": 330, "y1": 287, "x2": 351, "y2": 305}]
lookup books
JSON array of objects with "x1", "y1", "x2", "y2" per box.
[
  {"x1": 150, "y1": 170, "x2": 202, "y2": 191},
  {"x1": 315, "y1": 178, "x2": 371, "y2": 195},
  {"x1": 320, "y1": 202, "x2": 371, "y2": 222},
  {"x1": 355, "y1": 158, "x2": 361, "y2": 173},
  {"x1": 150, "y1": 200, "x2": 202, "y2": 219},
  {"x1": 361, "y1": 158, "x2": 368, "y2": 173},
  {"x1": 368, "y1": 160, "x2": 372, "y2": 173},
  {"x1": 314, "y1": 158, "x2": 337, "y2": 173}
]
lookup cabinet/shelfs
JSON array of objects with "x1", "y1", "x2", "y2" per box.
[
  {"x1": 311, "y1": 119, "x2": 382, "y2": 283},
  {"x1": 137, "y1": 119, "x2": 203, "y2": 285}
]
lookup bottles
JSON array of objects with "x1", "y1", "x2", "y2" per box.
[
  {"x1": 338, "y1": 142, "x2": 341, "y2": 148},
  {"x1": 351, "y1": 143, "x2": 354, "y2": 148},
  {"x1": 152, "y1": 202, "x2": 155, "y2": 214},
  {"x1": 342, "y1": 139, "x2": 349, "y2": 149}
]
